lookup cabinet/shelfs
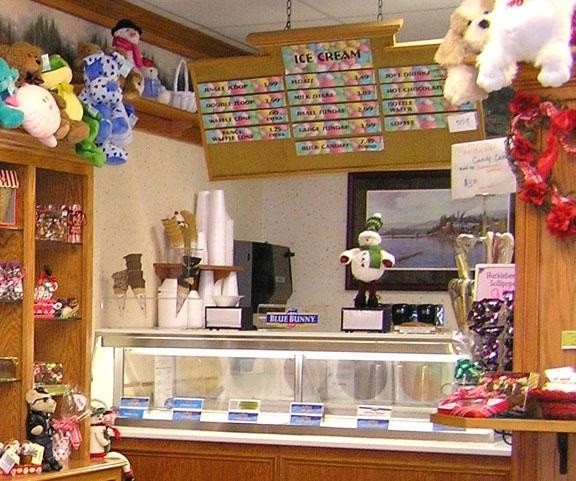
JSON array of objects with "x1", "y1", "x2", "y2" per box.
[
  {"x1": 28, "y1": 127, "x2": 92, "y2": 468},
  {"x1": 0, "y1": 127, "x2": 28, "y2": 443},
  {"x1": 108, "y1": 438, "x2": 510, "y2": 480},
  {"x1": 506, "y1": 59, "x2": 575, "y2": 481}
]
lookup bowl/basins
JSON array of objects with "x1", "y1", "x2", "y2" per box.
[{"x1": 155, "y1": 278, "x2": 202, "y2": 329}]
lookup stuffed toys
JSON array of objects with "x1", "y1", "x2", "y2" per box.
[
  {"x1": 0, "y1": 20, "x2": 172, "y2": 168},
  {"x1": 433, "y1": 1, "x2": 497, "y2": 107},
  {"x1": 475, "y1": 0, "x2": 573, "y2": 93},
  {"x1": 26, "y1": 388, "x2": 62, "y2": 472}
]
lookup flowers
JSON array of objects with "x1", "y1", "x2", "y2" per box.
[{"x1": 505, "y1": 91, "x2": 576, "y2": 243}]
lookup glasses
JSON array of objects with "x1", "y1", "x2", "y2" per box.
[{"x1": 468, "y1": 292, "x2": 513, "y2": 372}]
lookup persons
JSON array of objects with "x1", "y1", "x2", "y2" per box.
[
  {"x1": 336, "y1": 213, "x2": 396, "y2": 309},
  {"x1": 88, "y1": 407, "x2": 136, "y2": 481}
]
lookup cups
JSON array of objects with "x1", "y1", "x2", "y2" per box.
[
  {"x1": 382, "y1": 305, "x2": 445, "y2": 328},
  {"x1": 198, "y1": 272, "x2": 241, "y2": 329},
  {"x1": 190, "y1": 188, "x2": 235, "y2": 269}
]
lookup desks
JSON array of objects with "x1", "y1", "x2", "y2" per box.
[{"x1": 428, "y1": 412, "x2": 576, "y2": 480}]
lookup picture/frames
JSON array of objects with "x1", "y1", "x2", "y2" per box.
[{"x1": 344, "y1": 167, "x2": 515, "y2": 293}]
